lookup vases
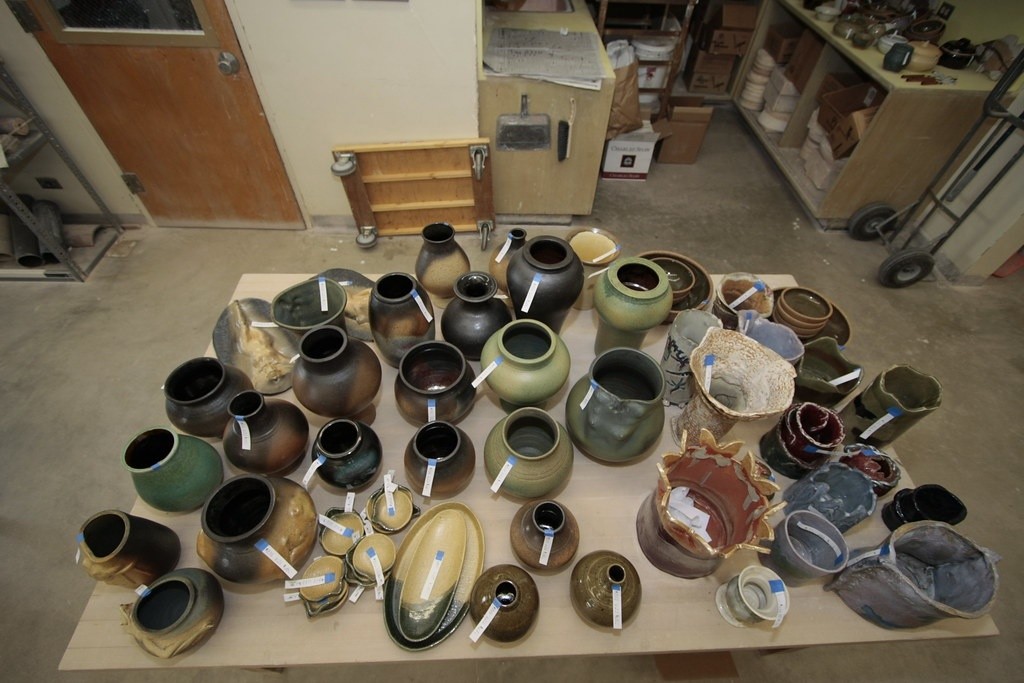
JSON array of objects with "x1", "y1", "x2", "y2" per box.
[{"x1": 76, "y1": 222, "x2": 1002, "y2": 661}]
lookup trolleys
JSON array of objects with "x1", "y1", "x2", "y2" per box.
[{"x1": 848, "y1": 48, "x2": 1024, "y2": 287}]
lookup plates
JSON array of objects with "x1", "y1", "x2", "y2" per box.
[
  {"x1": 382, "y1": 500, "x2": 487, "y2": 652},
  {"x1": 320, "y1": 480, "x2": 420, "y2": 588},
  {"x1": 298, "y1": 556, "x2": 349, "y2": 620},
  {"x1": 396, "y1": 509, "x2": 467, "y2": 643}
]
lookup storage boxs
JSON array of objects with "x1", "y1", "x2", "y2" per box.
[
  {"x1": 654, "y1": 96, "x2": 715, "y2": 164},
  {"x1": 758, "y1": 21, "x2": 889, "y2": 192},
  {"x1": 600, "y1": 113, "x2": 674, "y2": 183}
]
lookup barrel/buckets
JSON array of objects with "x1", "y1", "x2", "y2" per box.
[{"x1": 630, "y1": 19, "x2": 682, "y2": 106}]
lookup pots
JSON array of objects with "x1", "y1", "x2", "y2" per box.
[
  {"x1": 939, "y1": 37, "x2": 976, "y2": 69},
  {"x1": 909, "y1": 38, "x2": 942, "y2": 71}
]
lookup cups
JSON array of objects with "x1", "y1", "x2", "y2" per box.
[
  {"x1": 660, "y1": 309, "x2": 723, "y2": 404},
  {"x1": 882, "y1": 41, "x2": 915, "y2": 73},
  {"x1": 783, "y1": 462, "x2": 876, "y2": 527},
  {"x1": 827, "y1": 519, "x2": 1003, "y2": 630},
  {"x1": 565, "y1": 225, "x2": 622, "y2": 310},
  {"x1": 713, "y1": 271, "x2": 774, "y2": 331},
  {"x1": 391, "y1": 340, "x2": 478, "y2": 426},
  {"x1": 69, "y1": 507, "x2": 181, "y2": 590},
  {"x1": 842, "y1": 362, "x2": 945, "y2": 452},
  {"x1": 793, "y1": 336, "x2": 866, "y2": 409},
  {"x1": 880, "y1": 482, "x2": 968, "y2": 534},
  {"x1": 269, "y1": 276, "x2": 347, "y2": 340},
  {"x1": 715, "y1": 565, "x2": 790, "y2": 628},
  {"x1": 757, "y1": 510, "x2": 850, "y2": 588}
]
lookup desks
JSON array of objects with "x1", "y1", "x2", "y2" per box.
[{"x1": 57, "y1": 272, "x2": 1000, "y2": 674}]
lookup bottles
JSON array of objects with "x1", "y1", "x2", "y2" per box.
[
  {"x1": 565, "y1": 347, "x2": 666, "y2": 462},
  {"x1": 413, "y1": 221, "x2": 471, "y2": 299},
  {"x1": 162, "y1": 355, "x2": 256, "y2": 439},
  {"x1": 506, "y1": 234, "x2": 585, "y2": 335},
  {"x1": 593, "y1": 257, "x2": 674, "y2": 331},
  {"x1": 569, "y1": 549, "x2": 642, "y2": 627},
  {"x1": 194, "y1": 474, "x2": 319, "y2": 589},
  {"x1": 223, "y1": 389, "x2": 311, "y2": 474},
  {"x1": 440, "y1": 270, "x2": 513, "y2": 361},
  {"x1": 402, "y1": 420, "x2": 475, "y2": 497},
  {"x1": 488, "y1": 227, "x2": 530, "y2": 291},
  {"x1": 119, "y1": 426, "x2": 223, "y2": 512},
  {"x1": 291, "y1": 325, "x2": 381, "y2": 417},
  {"x1": 509, "y1": 499, "x2": 580, "y2": 572},
  {"x1": 479, "y1": 318, "x2": 571, "y2": 406},
  {"x1": 469, "y1": 563, "x2": 541, "y2": 642},
  {"x1": 482, "y1": 404, "x2": 573, "y2": 500},
  {"x1": 119, "y1": 569, "x2": 225, "y2": 662},
  {"x1": 367, "y1": 270, "x2": 436, "y2": 364},
  {"x1": 312, "y1": 417, "x2": 384, "y2": 487}
]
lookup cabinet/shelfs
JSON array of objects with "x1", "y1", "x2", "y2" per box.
[
  {"x1": 733, "y1": 0, "x2": 1015, "y2": 220},
  {"x1": 0, "y1": 62, "x2": 125, "y2": 283},
  {"x1": 479, "y1": 0, "x2": 617, "y2": 217},
  {"x1": 669, "y1": 0, "x2": 770, "y2": 100},
  {"x1": 588, "y1": 0, "x2": 696, "y2": 118}
]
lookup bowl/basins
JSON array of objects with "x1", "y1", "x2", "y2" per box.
[
  {"x1": 648, "y1": 257, "x2": 696, "y2": 304},
  {"x1": 814, "y1": 6, "x2": 841, "y2": 21},
  {"x1": 632, "y1": 250, "x2": 713, "y2": 323},
  {"x1": 772, "y1": 286, "x2": 833, "y2": 340},
  {"x1": 767, "y1": 287, "x2": 853, "y2": 351},
  {"x1": 911, "y1": 18, "x2": 943, "y2": 36}
]
